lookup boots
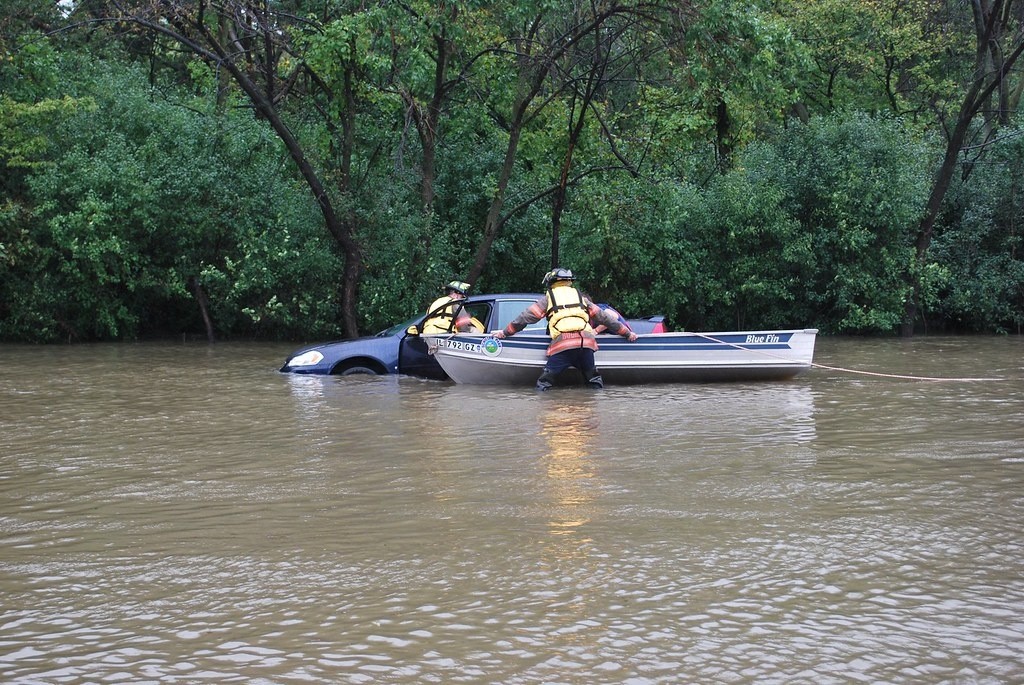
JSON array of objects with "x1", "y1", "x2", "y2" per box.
[
  {"x1": 536, "y1": 380, "x2": 553, "y2": 391},
  {"x1": 586, "y1": 375, "x2": 603, "y2": 389}
]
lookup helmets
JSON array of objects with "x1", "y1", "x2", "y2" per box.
[
  {"x1": 446, "y1": 281, "x2": 471, "y2": 294},
  {"x1": 542, "y1": 268, "x2": 577, "y2": 286}
]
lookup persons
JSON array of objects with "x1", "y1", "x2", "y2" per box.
[
  {"x1": 582, "y1": 292, "x2": 632, "y2": 336},
  {"x1": 423, "y1": 280, "x2": 481, "y2": 334},
  {"x1": 491, "y1": 267, "x2": 638, "y2": 392}
]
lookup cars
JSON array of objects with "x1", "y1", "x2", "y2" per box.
[{"x1": 278, "y1": 293, "x2": 668, "y2": 377}]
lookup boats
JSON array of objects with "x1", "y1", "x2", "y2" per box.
[{"x1": 419, "y1": 328, "x2": 818, "y2": 386}]
